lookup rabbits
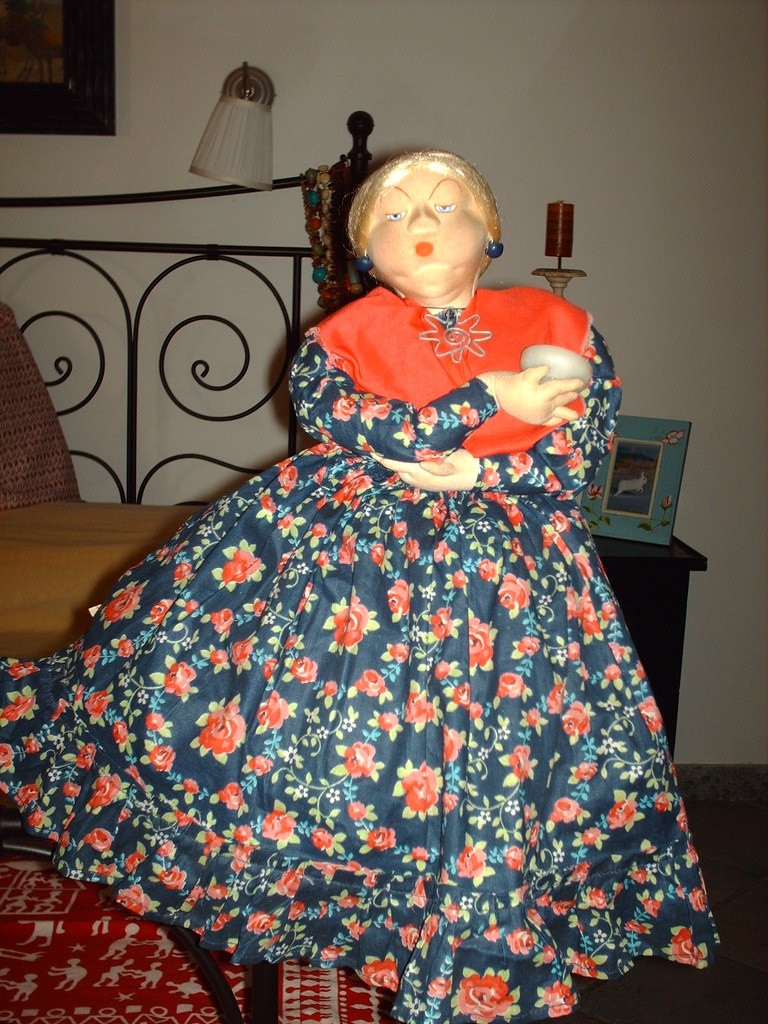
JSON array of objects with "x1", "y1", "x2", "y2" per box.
[{"x1": 613, "y1": 472, "x2": 647, "y2": 497}]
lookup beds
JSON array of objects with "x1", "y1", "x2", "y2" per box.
[{"x1": 1, "y1": 110, "x2": 396, "y2": 1024}]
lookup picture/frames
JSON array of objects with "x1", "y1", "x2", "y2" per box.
[
  {"x1": 580, "y1": 415, "x2": 692, "y2": 546},
  {"x1": 0, "y1": 0, "x2": 117, "y2": 136}
]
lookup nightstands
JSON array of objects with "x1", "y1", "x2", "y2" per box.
[{"x1": 593, "y1": 534, "x2": 708, "y2": 761}]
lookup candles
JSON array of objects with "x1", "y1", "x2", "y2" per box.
[{"x1": 545, "y1": 200, "x2": 575, "y2": 258}]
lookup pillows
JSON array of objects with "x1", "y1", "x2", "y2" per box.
[{"x1": 0, "y1": 302, "x2": 81, "y2": 513}]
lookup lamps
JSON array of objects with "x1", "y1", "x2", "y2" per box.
[{"x1": 189, "y1": 59, "x2": 277, "y2": 191}]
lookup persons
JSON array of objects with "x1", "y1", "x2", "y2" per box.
[{"x1": 0, "y1": 149, "x2": 719, "y2": 1024}]
